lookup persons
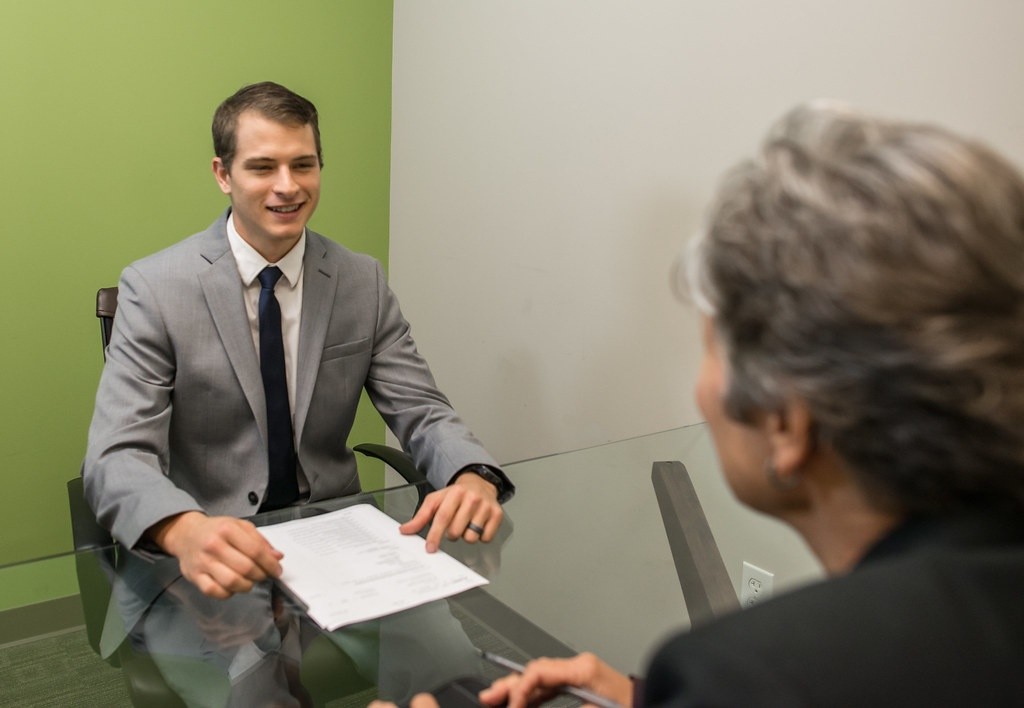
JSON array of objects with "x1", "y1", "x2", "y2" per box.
[
  {"x1": 368, "y1": 106, "x2": 1024, "y2": 707},
  {"x1": 82, "y1": 82, "x2": 515, "y2": 707}
]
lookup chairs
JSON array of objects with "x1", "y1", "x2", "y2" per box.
[{"x1": 66, "y1": 285, "x2": 429, "y2": 708}]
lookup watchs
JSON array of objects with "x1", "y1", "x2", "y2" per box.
[{"x1": 468, "y1": 463, "x2": 504, "y2": 496}]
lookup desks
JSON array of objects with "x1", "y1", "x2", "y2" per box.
[{"x1": 0, "y1": 420, "x2": 823, "y2": 708}]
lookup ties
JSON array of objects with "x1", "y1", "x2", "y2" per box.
[{"x1": 255, "y1": 265, "x2": 301, "y2": 515}]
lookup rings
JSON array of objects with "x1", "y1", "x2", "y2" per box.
[{"x1": 468, "y1": 522, "x2": 483, "y2": 533}]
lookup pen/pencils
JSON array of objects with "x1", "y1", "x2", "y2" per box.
[{"x1": 469, "y1": 645, "x2": 625, "y2": 708}]
